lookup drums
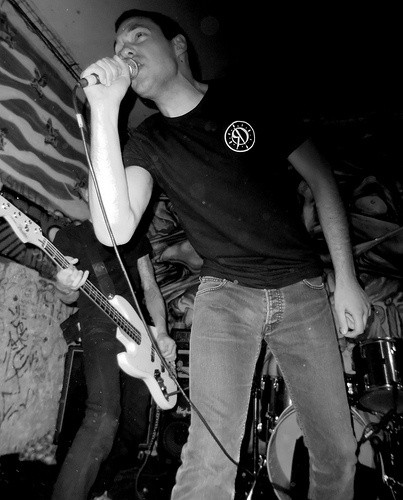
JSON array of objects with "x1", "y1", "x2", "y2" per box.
[
  {"x1": 268, "y1": 402, "x2": 403, "y2": 499},
  {"x1": 349, "y1": 334, "x2": 403, "y2": 420},
  {"x1": 259, "y1": 372, "x2": 294, "y2": 445}
]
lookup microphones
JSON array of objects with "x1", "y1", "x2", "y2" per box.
[{"x1": 75, "y1": 58, "x2": 139, "y2": 88}]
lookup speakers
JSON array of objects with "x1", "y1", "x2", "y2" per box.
[
  {"x1": 154, "y1": 351, "x2": 189, "y2": 464},
  {"x1": 54, "y1": 344, "x2": 154, "y2": 449}
]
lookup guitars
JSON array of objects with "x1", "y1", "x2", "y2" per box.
[{"x1": 1, "y1": 195, "x2": 177, "y2": 411}]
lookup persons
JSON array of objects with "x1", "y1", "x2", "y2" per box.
[
  {"x1": 75, "y1": 8, "x2": 375, "y2": 500},
  {"x1": 53, "y1": 213, "x2": 177, "y2": 500}
]
